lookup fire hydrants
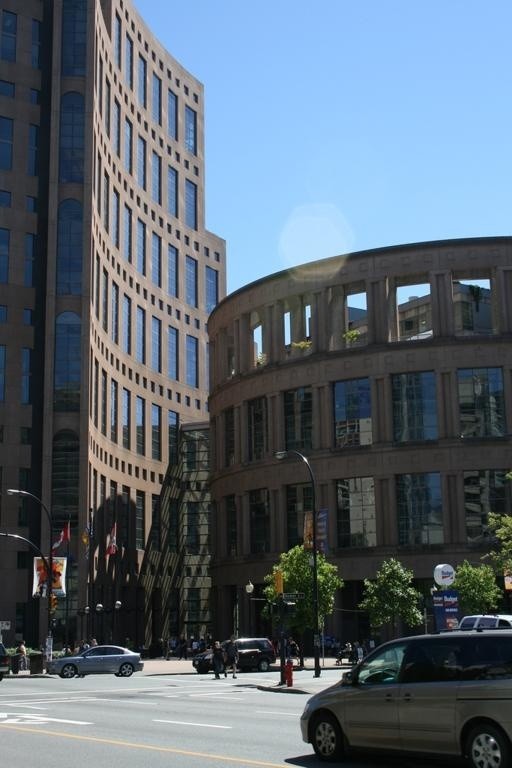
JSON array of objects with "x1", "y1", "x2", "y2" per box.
[{"x1": 283, "y1": 656, "x2": 294, "y2": 687}]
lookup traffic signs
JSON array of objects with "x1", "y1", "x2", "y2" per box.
[{"x1": 282, "y1": 593, "x2": 305, "y2": 600}]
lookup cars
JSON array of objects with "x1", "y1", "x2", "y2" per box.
[
  {"x1": 0, "y1": 639, "x2": 9, "y2": 682},
  {"x1": 45, "y1": 645, "x2": 144, "y2": 679}
]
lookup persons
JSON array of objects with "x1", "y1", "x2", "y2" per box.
[
  {"x1": 16, "y1": 639, "x2": 28, "y2": 670},
  {"x1": 333, "y1": 638, "x2": 370, "y2": 667},
  {"x1": 158, "y1": 632, "x2": 300, "y2": 681},
  {"x1": 58, "y1": 637, "x2": 98, "y2": 679},
  {"x1": 124, "y1": 637, "x2": 132, "y2": 650},
  {"x1": 137, "y1": 640, "x2": 150, "y2": 659}
]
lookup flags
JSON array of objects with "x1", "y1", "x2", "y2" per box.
[
  {"x1": 81, "y1": 523, "x2": 93, "y2": 558},
  {"x1": 104, "y1": 522, "x2": 118, "y2": 561},
  {"x1": 51, "y1": 518, "x2": 71, "y2": 556}
]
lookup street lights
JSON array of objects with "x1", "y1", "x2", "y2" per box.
[
  {"x1": 79, "y1": 609, "x2": 84, "y2": 640},
  {"x1": 84, "y1": 604, "x2": 92, "y2": 644},
  {"x1": 245, "y1": 580, "x2": 254, "y2": 638},
  {"x1": 95, "y1": 600, "x2": 105, "y2": 646},
  {"x1": 274, "y1": 450, "x2": 321, "y2": 677},
  {"x1": 8, "y1": 488, "x2": 54, "y2": 675},
  {"x1": 114, "y1": 599, "x2": 122, "y2": 644}
]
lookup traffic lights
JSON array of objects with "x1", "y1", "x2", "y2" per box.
[{"x1": 273, "y1": 569, "x2": 284, "y2": 594}]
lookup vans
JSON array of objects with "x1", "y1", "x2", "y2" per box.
[
  {"x1": 457, "y1": 613, "x2": 511, "y2": 631},
  {"x1": 193, "y1": 637, "x2": 276, "y2": 674},
  {"x1": 300, "y1": 627, "x2": 511, "y2": 768}
]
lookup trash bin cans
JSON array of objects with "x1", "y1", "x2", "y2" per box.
[{"x1": 29, "y1": 651, "x2": 43, "y2": 674}]
